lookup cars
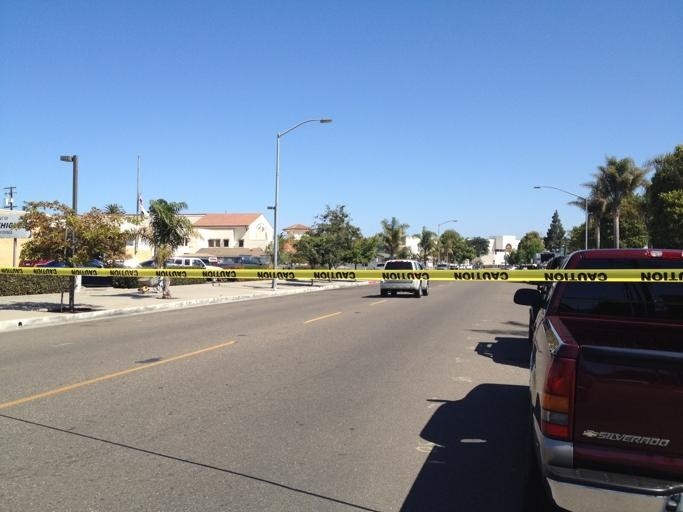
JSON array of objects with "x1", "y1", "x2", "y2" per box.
[
  {"x1": 502, "y1": 264, "x2": 518, "y2": 271},
  {"x1": 437, "y1": 263, "x2": 473, "y2": 270},
  {"x1": 375, "y1": 259, "x2": 429, "y2": 297},
  {"x1": 19, "y1": 256, "x2": 267, "y2": 269},
  {"x1": 530, "y1": 254, "x2": 565, "y2": 340}
]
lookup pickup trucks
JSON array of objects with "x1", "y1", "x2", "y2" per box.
[{"x1": 514, "y1": 248, "x2": 683, "y2": 511}]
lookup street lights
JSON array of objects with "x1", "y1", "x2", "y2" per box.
[
  {"x1": 438, "y1": 219, "x2": 458, "y2": 263},
  {"x1": 61, "y1": 154, "x2": 78, "y2": 313},
  {"x1": 267, "y1": 119, "x2": 332, "y2": 288},
  {"x1": 534, "y1": 186, "x2": 588, "y2": 247}
]
